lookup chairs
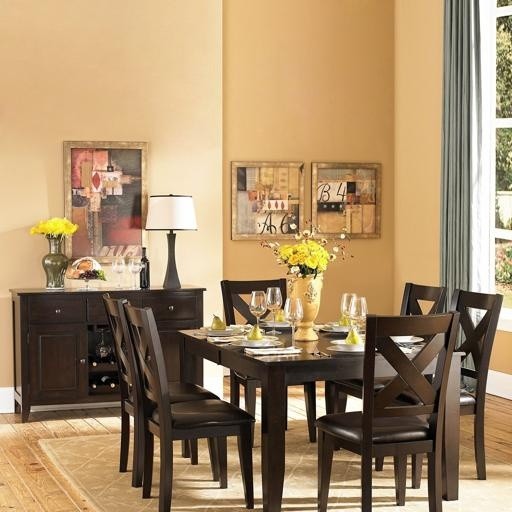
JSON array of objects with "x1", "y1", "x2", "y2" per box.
[
  {"x1": 322, "y1": 281, "x2": 447, "y2": 452},
  {"x1": 220, "y1": 277, "x2": 318, "y2": 449},
  {"x1": 313, "y1": 310, "x2": 460, "y2": 510},
  {"x1": 367, "y1": 289, "x2": 505, "y2": 487},
  {"x1": 122, "y1": 300, "x2": 256, "y2": 511},
  {"x1": 102, "y1": 290, "x2": 223, "y2": 487}
]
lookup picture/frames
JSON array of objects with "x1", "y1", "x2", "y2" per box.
[
  {"x1": 311, "y1": 162, "x2": 384, "y2": 240},
  {"x1": 62, "y1": 139, "x2": 149, "y2": 267},
  {"x1": 230, "y1": 160, "x2": 305, "y2": 242}
]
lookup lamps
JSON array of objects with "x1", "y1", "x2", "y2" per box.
[{"x1": 143, "y1": 193, "x2": 200, "y2": 289}]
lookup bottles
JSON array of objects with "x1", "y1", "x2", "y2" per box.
[
  {"x1": 139, "y1": 246, "x2": 151, "y2": 288},
  {"x1": 95, "y1": 328, "x2": 110, "y2": 362},
  {"x1": 89, "y1": 374, "x2": 116, "y2": 389},
  {"x1": 108, "y1": 352, "x2": 115, "y2": 365},
  {"x1": 88, "y1": 353, "x2": 98, "y2": 367}
]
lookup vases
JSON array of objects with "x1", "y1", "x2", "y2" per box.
[
  {"x1": 288, "y1": 277, "x2": 322, "y2": 341},
  {"x1": 42, "y1": 232, "x2": 69, "y2": 290}
]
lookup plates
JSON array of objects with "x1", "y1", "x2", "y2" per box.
[
  {"x1": 331, "y1": 339, "x2": 365, "y2": 344},
  {"x1": 197, "y1": 332, "x2": 229, "y2": 337},
  {"x1": 233, "y1": 336, "x2": 278, "y2": 341},
  {"x1": 260, "y1": 323, "x2": 292, "y2": 330},
  {"x1": 231, "y1": 340, "x2": 282, "y2": 347},
  {"x1": 327, "y1": 346, "x2": 378, "y2": 356},
  {"x1": 392, "y1": 336, "x2": 425, "y2": 344},
  {"x1": 319, "y1": 325, "x2": 348, "y2": 333}
]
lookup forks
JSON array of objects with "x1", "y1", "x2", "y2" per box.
[{"x1": 305, "y1": 347, "x2": 331, "y2": 357}]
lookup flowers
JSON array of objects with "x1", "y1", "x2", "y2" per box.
[
  {"x1": 29, "y1": 214, "x2": 80, "y2": 244},
  {"x1": 260, "y1": 218, "x2": 354, "y2": 276}
]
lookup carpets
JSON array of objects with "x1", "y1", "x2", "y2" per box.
[{"x1": 26, "y1": 418, "x2": 451, "y2": 510}]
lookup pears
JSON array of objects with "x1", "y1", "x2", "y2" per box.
[
  {"x1": 339, "y1": 314, "x2": 349, "y2": 326},
  {"x1": 247, "y1": 324, "x2": 262, "y2": 340},
  {"x1": 211, "y1": 314, "x2": 226, "y2": 331},
  {"x1": 346, "y1": 329, "x2": 359, "y2": 345},
  {"x1": 275, "y1": 312, "x2": 284, "y2": 322}
]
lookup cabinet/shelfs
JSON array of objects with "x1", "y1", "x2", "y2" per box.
[
  {"x1": 86, "y1": 289, "x2": 140, "y2": 405},
  {"x1": 11, "y1": 289, "x2": 88, "y2": 404},
  {"x1": 140, "y1": 289, "x2": 204, "y2": 392}
]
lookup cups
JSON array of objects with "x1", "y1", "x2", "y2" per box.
[{"x1": 230, "y1": 324, "x2": 244, "y2": 336}]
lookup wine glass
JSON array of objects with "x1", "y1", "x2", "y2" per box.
[
  {"x1": 112, "y1": 255, "x2": 126, "y2": 288},
  {"x1": 266, "y1": 287, "x2": 283, "y2": 335},
  {"x1": 348, "y1": 297, "x2": 369, "y2": 340},
  {"x1": 282, "y1": 297, "x2": 303, "y2": 351},
  {"x1": 129, "y1": 259, "x2": 142, "y2": 290},
  {"x1": 340, "y1": 292, "x2": 356, "y2": 326},
  {"x1": 249, "y1": 291, "x2": 266, "y2": 332}
]
